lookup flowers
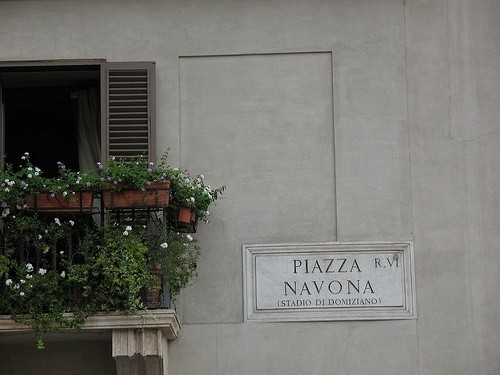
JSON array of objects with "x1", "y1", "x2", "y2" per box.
[
  {"x1": 0, "y1": 222, "x2": 199, "y2": 350},
  {"x1": 91, "y1": 147, "x2": 176, "y2": 192},
  {"x1": 1, "y1": 151, "x2": 101, "y2": 219},
  {"x1": 168, "y1": 166, "x2": 227, "y2": 224}
]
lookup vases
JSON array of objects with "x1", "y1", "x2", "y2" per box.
[
  {"x1": 138, "y1": 275, "x2": 161, "y2": 309},
  {"x1": 100, "y1": 179, "x2": 170, "y2": 210},
  {"x1": 1, "y1": 188, "x2": 94, "y2": 214},
  {"x1": 171, "y1": 201, "x2": 196, "y2": 226}
]
